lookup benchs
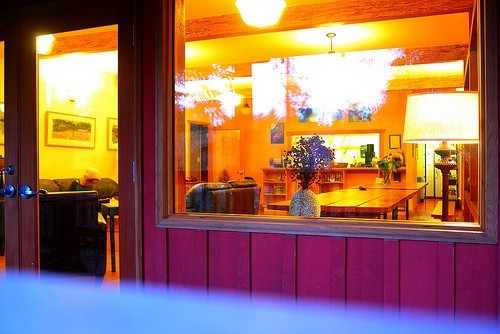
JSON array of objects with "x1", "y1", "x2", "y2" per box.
[{"x1": 431, "y1": 201, "x2": 455, "y2": 219}]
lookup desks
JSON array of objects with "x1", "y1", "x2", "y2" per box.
[
  {"x1": 266, "y1": 182, "x2": 428, "y2": 220},
  {"x1": 102, "y1": 203, "x2": 119, "y2": 272}
]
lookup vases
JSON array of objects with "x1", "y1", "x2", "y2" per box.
[
  {"x1": 289, "y1": 189, "x2": 320, "y2": 218},
  {"x1": 382, "y1": 169, "x2": 392, "y2": 185}
]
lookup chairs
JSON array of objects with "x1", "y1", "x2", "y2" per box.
[{"x1": 39, "y1": 190, "x2": 107, "y2": 288}]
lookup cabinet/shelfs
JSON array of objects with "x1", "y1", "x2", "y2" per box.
[{"x1": 260, "y1": 167, "x2": 406, "y2": 217}]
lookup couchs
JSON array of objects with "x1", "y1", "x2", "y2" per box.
[
  {"x1": 186, "y1": 180, "x2": 261, "y2": 215},
  {"x1": 39, "y1": 178, "x2": 119, "y2": 198}
]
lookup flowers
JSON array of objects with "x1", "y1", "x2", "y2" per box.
[
  {"x1": 372, "y1": 152, "x2": 403, "y2": 183},
  {"x1": 282, "y1": 132, "x2": 336, "y2": 190}
]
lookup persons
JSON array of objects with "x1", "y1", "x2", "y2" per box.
[{"x1": 68, "y1": 168, "x2": 99, "y2": 191}]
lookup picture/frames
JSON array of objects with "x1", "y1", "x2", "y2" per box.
[
  {"x1": 0, "y1": 119, "x2": 4, "y2": 145},
  {"x1": 44, "y1": 111, "x2": 96, "y2": 149},
  {"x1": 107, "y1": 117, "x2": 118, "y2": 150}
]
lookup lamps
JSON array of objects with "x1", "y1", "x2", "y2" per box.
[{"x1": 403, "y1": 92, "x2": 480, "y2": 221}]
